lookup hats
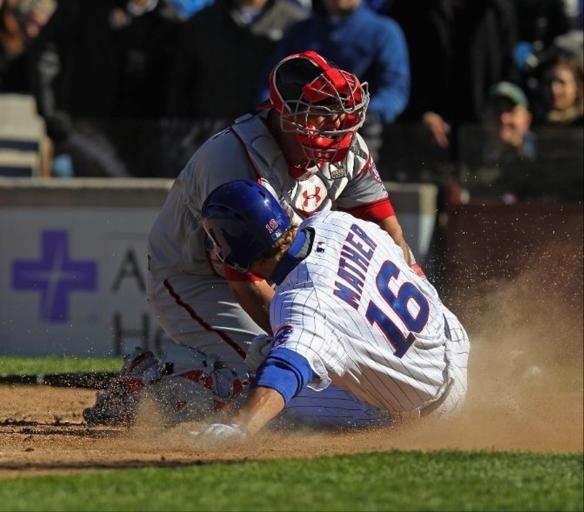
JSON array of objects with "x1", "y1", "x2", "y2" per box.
[{"x1": 486, "y1": 80, "x2": 528, "y2": 108}]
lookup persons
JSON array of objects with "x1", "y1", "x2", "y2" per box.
[
  {"x1": 80, "y1": 47, "x2": 444, "y2": 430},
  {"x1": 0, "y1": 2, "x2": 583, "y2": 207},
  {"x1": 188, "y1": 178, "x2": 471, "y2": 451}
]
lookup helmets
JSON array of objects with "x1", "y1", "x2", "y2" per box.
[
  {"x1": 267, "y1": 48, "x2": 362, "y2": 128},
  {"x1": 199, "y1": 177, "x2": 295, "y2": 267}
]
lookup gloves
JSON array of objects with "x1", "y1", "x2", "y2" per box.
[{"x1": 186, "y1": 422, "x2": 253, "y2": 451}]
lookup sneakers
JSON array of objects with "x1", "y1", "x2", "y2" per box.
[{"x1": 83, "y1": 350, "x2": 164, "y2": 426}]
